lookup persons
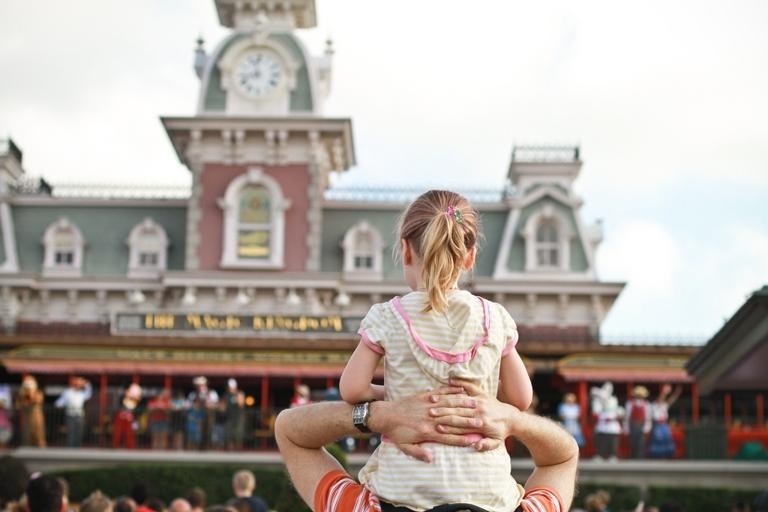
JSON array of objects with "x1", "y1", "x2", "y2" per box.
[
  {"x1": 519, "y1": 380, "x2": 766, "y2": 511},
  {"x1": 0, "y1": 372, "x2": 336, "y2": 511},
  {"x1": 336, "y1": 190, "x2": 535, "y2": 512},
  {"x1": 272, "y1": 377, "x2": 579, "y2": 510}
]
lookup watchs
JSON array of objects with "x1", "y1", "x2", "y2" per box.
[{"x1": 352, "y1": 399, "x2": 373, "y2": 435}]
